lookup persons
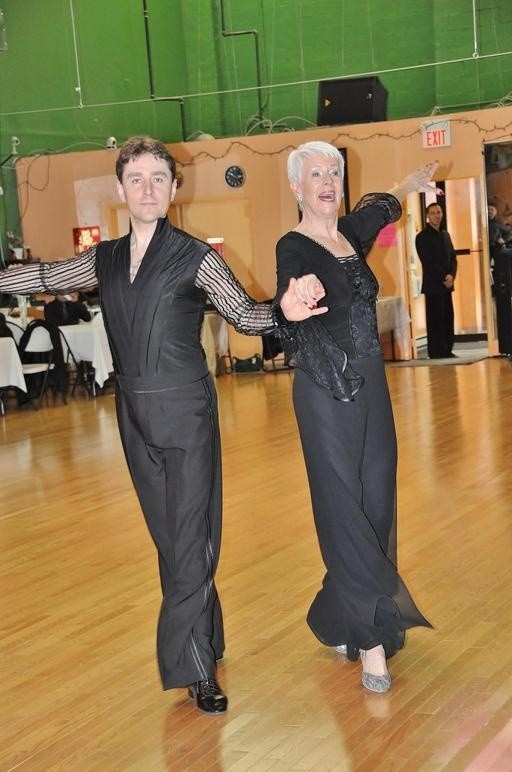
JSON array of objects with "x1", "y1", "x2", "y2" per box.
[
  {"x1": 44, "y1": 291, "x2": 91, "y2": 325},
  {"x1": 488, "y1": 194, "x2": 512, "y2": 250},
  {"x1": 275, "y1": 141, "x2": 445, "y2": 693},
  {"x1": 416, "y1": 203, "x2": 457, "y2": 358},
  {"x1": 0, "y1": 136, "x2": 329, "y2": 712}
]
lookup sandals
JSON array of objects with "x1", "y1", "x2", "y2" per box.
[{"x1": 335, "y1": 644, "x2": 392, "y2": 693}]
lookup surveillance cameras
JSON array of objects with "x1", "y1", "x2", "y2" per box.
[
  {"x1": 106, "y1": 136, "x2": 117, "y2": 148},
  {"x1": 11, "y1": 135, "x2": 20, "y2": 154}
]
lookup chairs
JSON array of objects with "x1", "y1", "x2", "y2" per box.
[{"x1": 1, "y1": 321, "x2": 92, "y2": 413}]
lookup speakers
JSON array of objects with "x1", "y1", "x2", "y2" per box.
[{"x1": 316, "y1": 74, "x2": 390, "y2": 125}]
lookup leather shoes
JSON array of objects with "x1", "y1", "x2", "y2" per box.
[
  {"x1": 429, "y1": 353, "x2": 455, "y2": 359},
  {"x1": 188, "y1": 654, "x2": 228, "y2": 715}
]
